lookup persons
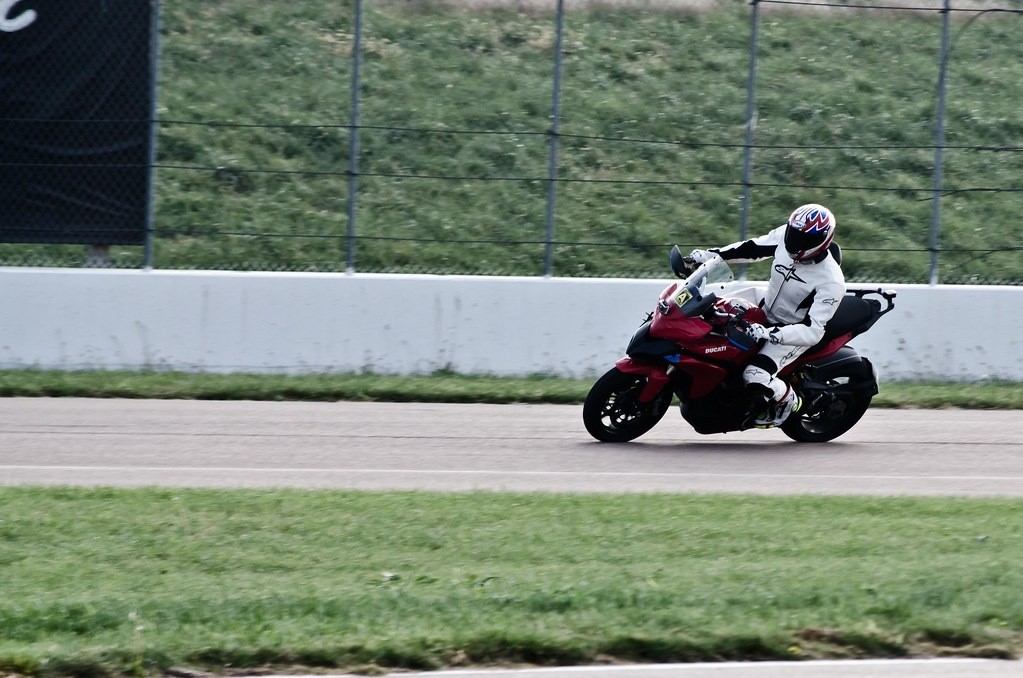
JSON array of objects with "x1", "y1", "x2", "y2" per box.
[{"x1": 690, "y1": 204, "x2": 846, "y2": 429}]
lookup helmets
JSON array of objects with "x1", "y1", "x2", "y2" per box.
[{"x1": 784, "y1": 203, "x2": 836, "y2": 264}]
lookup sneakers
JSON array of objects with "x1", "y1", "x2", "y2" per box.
[{"x1": 754, "y1": 382, "x2": 802, "y2": 430}]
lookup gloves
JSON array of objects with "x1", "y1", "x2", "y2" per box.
[
  {"x1": 683, "y1": 249, "x2": 719, "y2": 270},
  {"x1": 746, "y1": 323, "x2": 782, "y2": 345}
]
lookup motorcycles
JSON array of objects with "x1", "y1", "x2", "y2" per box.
[{"x1": 583, "y1": 245, "x2": 897, "y2": 442}]
